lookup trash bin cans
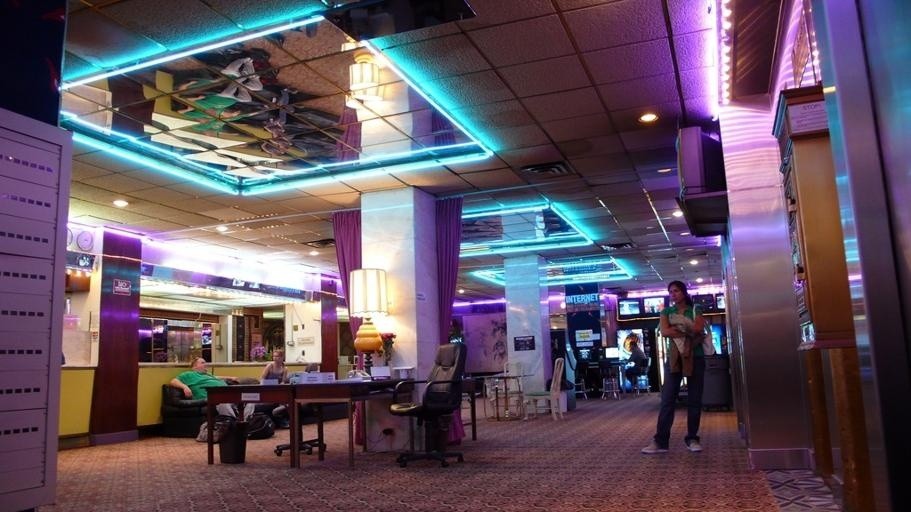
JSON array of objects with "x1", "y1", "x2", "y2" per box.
[
  {"x1": 701, "y1": 354, "x2": 731, "y2": 412},
  {"x1": 216, "y1": 421, "x2": 251, "y2": 464}
]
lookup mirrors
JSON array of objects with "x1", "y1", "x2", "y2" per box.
[{"x1": 139, "y1": 292, "x2": 286, "y2": 367}]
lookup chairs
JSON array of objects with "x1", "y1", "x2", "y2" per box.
[
  {"x1": 274, "y1": 406, "x2": 326, "y2": 457},
  {"x1": 574, "y1": 361, "x2": 594, "y2": 400},
  {"x1": 485, "y1": 357, "x2": 564, "y2": 422},
  {"x1": 633, "y1": 357, "x2": 652, "y2": 399},
  {"x1": 391, "y1": 343, "x2": 466, "y2": 468},
  {"x1": 598, "y1": 359, "x2": 621, "y2": 402}
]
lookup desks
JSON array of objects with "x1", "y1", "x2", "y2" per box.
[{"x1": 199, "y1": 379, "x2": 414, "y2": 467}]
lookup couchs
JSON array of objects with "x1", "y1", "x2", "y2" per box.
[{"x1": 161, "y1": 377, "x2": 260, "y2": 437}]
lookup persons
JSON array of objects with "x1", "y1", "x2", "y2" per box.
[
  {"x1": 625, "y1": 341, "x2": 647, "y2": 387},
  {"x1": 172, "y1": 56, "x2": 305, "y2": 158},
  {"x1": 641, "y1": 280, "x2": 706, "y2": 454},
  {"x1": 703, "y1": 332, "x2": 716, "y2": 356},
  {"x1": 169, "y1": 357, "x2": 237, "y2": 418},
  {"x1": 261, "y1": 349, "x2": 288, "y2": 385}
]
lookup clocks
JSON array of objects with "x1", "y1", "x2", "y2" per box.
[{"x1": 77, "y1": 231, "x2": 94, "y2": 251}]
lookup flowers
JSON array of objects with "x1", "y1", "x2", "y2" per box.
[
  {"x1": 250, "y1": 343, "x2": 272, "y2": 361},
  {"x1": 380, "y1": 332, "x2": 396, "y2": 365}
]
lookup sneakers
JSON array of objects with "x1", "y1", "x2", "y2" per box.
[
  {"x1": 641, "y1": 437, "x2": 669, "y2": 454},
  {"x1": 685, "y1": 436, "x2": 703, "y2": 452}
]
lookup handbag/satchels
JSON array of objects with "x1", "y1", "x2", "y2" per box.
[
  {"x1": 196, "y1": 420, "x2": 219, "y2": 444},
  {"x1": 245, "y1": 412, "x2": 274, "y2": 440},
  {"x1": 704, "y1": 333, "x2": 717, "y2": 360}
]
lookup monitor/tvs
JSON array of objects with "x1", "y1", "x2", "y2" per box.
[
  {"x1": 715, "y1": 293, "x2": 725, "y2": 312},
  {"x1": 618, "y1": 298, "x2": 641, "y2": 318},
  {"x1": 604, "y1": 347, "x2": 619, "y2": 359},
  {"x1": 643, "y1": 296, "x2": 669, "y2": 317},
  {"x1": 692, "y1": 294, "x2": 714, "y2": 313}
]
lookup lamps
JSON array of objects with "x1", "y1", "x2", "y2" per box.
[
  {"x1": 349, "y1": 268, "x2": 389, "y2": 378},
  {"x1": 345, "y1": 48, "x2": 383, "y2": 99}
]
